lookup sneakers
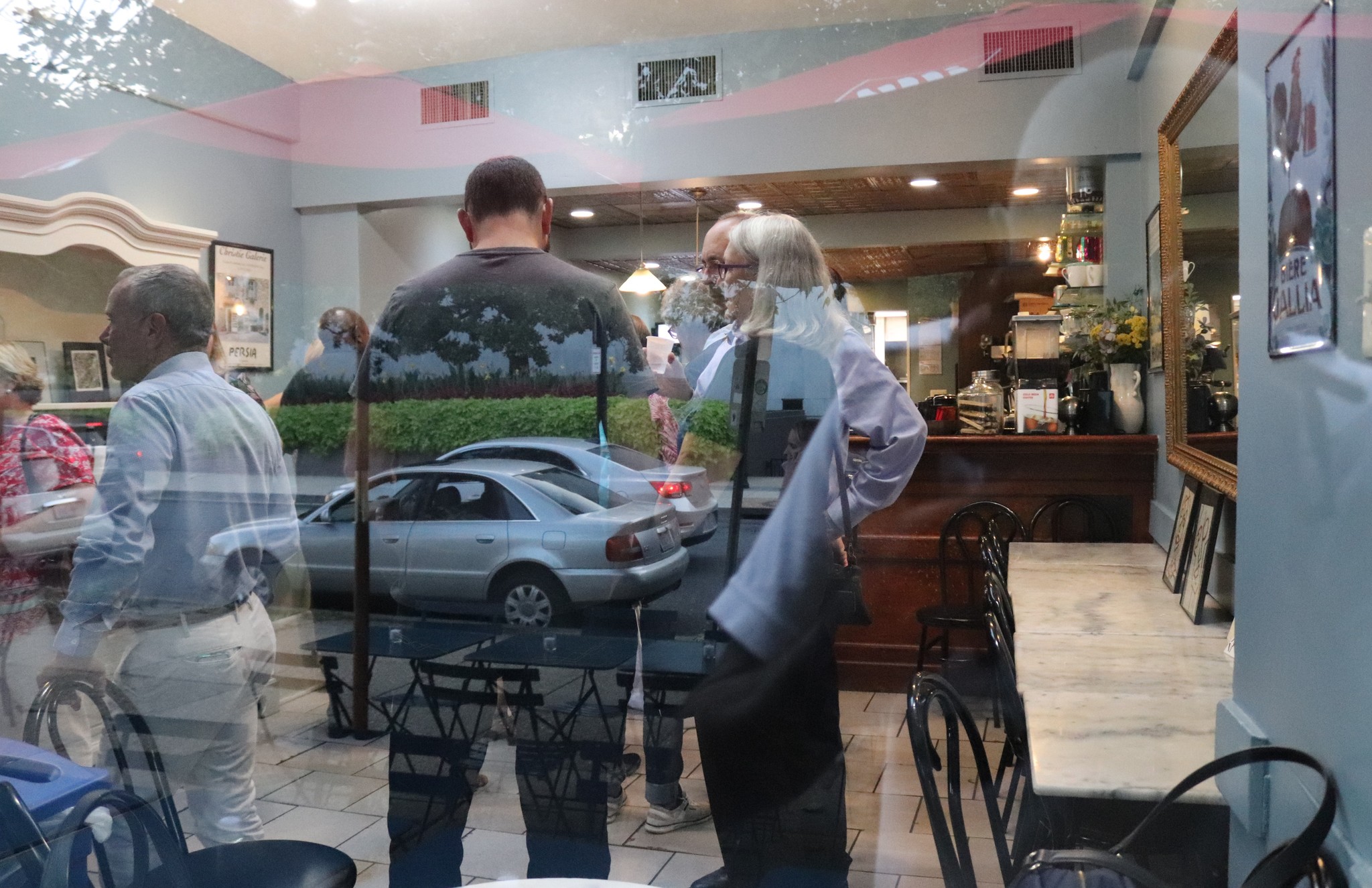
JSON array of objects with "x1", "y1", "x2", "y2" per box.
[
  {"x1": 606, "y1": 787, "x2": 628, "y2": 824},
  {"x1": 643, "y1": 791, "x2": 712, "y2": 834}
]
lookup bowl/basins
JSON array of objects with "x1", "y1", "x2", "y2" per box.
[{"x1": 1059, "y1": 323, "x2": 1069, "y2": 337}]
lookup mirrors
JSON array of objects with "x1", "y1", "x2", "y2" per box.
[{"x1": 1158, "y1": 11, "x2": 1239, "y2": 502}]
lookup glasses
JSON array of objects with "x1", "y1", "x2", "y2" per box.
[
  {"x1": 717, "y1": 260, "x2": 758, "y2": 279},
  {"x1": 694, "y1": 259, "x2": 722, "y2": 280},
  {"x1": 667, "y1": 324, "x2": 678, "y2": 339}
]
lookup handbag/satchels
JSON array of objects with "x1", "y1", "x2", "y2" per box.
[{"x1": 20, "y1": 407, "x2": 102, "y2": 626}]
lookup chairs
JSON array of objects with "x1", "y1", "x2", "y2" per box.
[
  {"x1": 374, "y1": 659, "x2": 689, "y2": 841},
  {"x1": 905, "y1": 498, "x2": 1135, "y2": 888},
  {"x1": 0, "y1": 672, "x2": 359, "y2": 888}
]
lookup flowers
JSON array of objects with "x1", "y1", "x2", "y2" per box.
[
  {"x1": 1068, "y1": 286, "x2": 1149, "y2": 370},
  {"x1": 1183, "y1": 283, "x2": 1215, "y2": 380}
]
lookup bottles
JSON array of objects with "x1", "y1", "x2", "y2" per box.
[
  {"x1": 1055, "y1": 214, "x2": 1103, "y2": 263},
  {"x1": 924, "y1": 369, "x2": 1004, "y2": 436}
]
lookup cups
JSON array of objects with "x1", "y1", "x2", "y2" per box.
[
  {"x1": 646, "y1": 336, "x2": 675, "y2": 374},
  {"x1": 1061, "y1": 264, "x2": 1103, "y2": 287},
  {"x1": 1182, "y1": 260, "x2": 1195, "y2": 282}
]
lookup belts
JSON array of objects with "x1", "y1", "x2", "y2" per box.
[{"x1": 125, "y1": 588, "x2": 253, "y2": 633}]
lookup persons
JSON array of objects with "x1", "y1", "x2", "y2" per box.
[
  {"x1": 0, "y1": 154, "x2": 930, "y2": 887},
  {"x1": 985, "y1": 45, "x2": 1372, "y2": 887}
]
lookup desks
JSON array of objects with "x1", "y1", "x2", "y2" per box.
[
  {"x1": 32, "y1": 401, "x2": 117, "y2": 416},
  {"x1": 1006, "y1": 542, "x2": 1229, "y2": 888},
  {"x1": 300, "y1": 625, "x2": 705, "y2": 755}
]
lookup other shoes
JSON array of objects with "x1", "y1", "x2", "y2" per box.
[{"x1": 622, "y1": 752, "x2": 641, "y2": 776}]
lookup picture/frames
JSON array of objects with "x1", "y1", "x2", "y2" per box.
[
  {"x1": 1145, "y1": 204, "x2": 1164, "y2": 373},
  {"x1": 62, "y1": 341, "x2": 111, "y2": 402},
  {"x1": 1162, "y1": 474, "x2": 1224, "y2": 593},
  {"x1": 209, "y1": 240, "x2": 275, "y2": 372}
]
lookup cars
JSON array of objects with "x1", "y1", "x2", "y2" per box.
[
  {"x1": 320, "y1": 437, "x2": 718, "y2": 547},
  {"x1": 0, "y1": 420, "x2": 144, "y2": 569},
  {"x1": 197, "y1": 462, "x2": 687, "y2": 630}
]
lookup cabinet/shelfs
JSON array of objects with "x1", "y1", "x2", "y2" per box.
[{"x1": 1051, "y1": 213, "x2": 1103, "y2": 307}]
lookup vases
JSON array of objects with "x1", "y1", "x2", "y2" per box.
[
  {"x1": 1188, "y1": 380, "x2": 1211, "y2": 433},
  {"x1": 1109, "y1": 364, "x2": 1144, "y2": 433}
]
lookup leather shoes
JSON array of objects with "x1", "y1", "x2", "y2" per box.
[{"x1": 689, "y1": 864, "x2": 732, "y2": 888}]
